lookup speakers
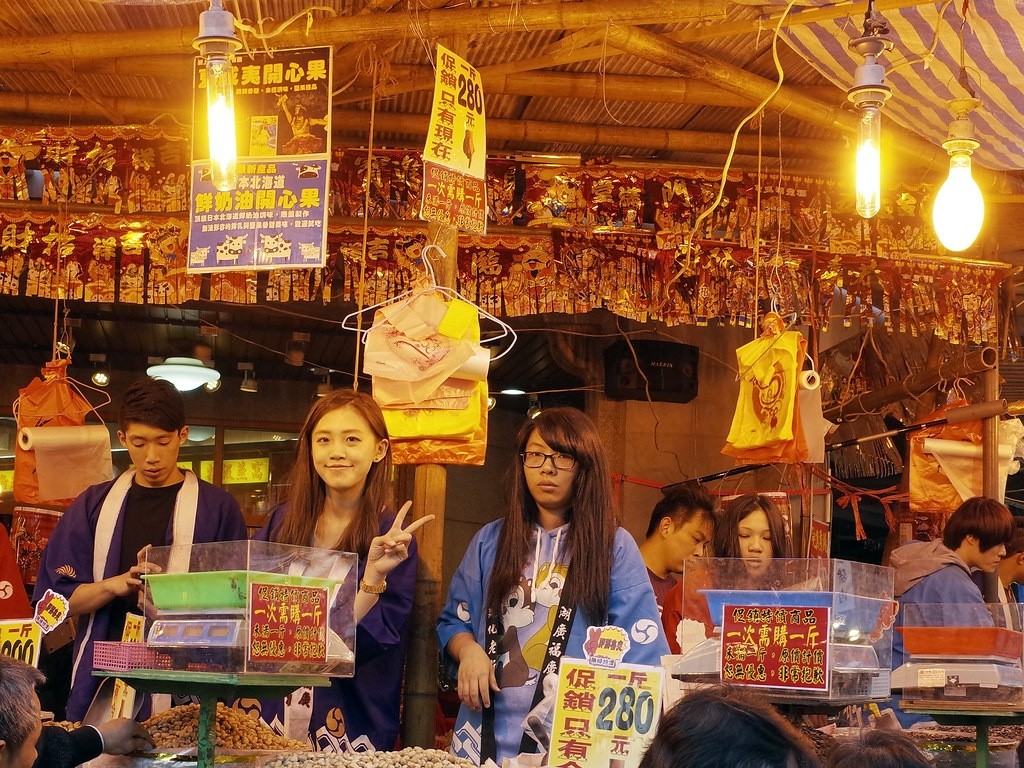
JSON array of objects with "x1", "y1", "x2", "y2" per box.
[{"x1": 605, "y1": 339, "x2": 699, "y2": 403}]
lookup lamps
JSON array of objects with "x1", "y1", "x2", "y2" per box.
[
  {"x1": 281, "y1": 330, "x2": 311, "y2": 367},
  {"x1": 237, "y1": 362, "x2": 258, "y2": 393},
  {"x1": 312, "y1": 368, "x2": 334, "y2": 397},
  {"x1": 88, "y1": 354, "x2": 110, "y2": 387},
  {"x1": 145, "y1": 356, "x2": 220, "y2": 391},
  {"x1": 527, "y1": 393, "x2": 541, "y2": 419}
]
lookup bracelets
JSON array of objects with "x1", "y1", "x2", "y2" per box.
[{"x1": 360, "y1": 579, "x2": 387, "y2": 593}]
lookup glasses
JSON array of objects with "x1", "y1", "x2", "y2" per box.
[{"x1": 520, "y1": 451, "x2": 579, "y2": 470}]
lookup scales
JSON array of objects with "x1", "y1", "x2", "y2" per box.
[
  {"x1": 671, "y1": 590, "x2": 892, "y2": 698},
  {"x1": 139, "y1": 569, "x2": 344, "y2": 671},
  {"x1": 892, "y1": 626, "x2": 1024, "y2": 700}
]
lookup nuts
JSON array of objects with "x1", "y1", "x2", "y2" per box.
[
  {"x1": 41, "y1": 720, "x2": 83, "y2": 734},
  {"x1": 133, "y1": 702, "x2": 477, "y2": 768}
]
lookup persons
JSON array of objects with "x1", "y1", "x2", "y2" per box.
[
  {"x1": 971, "y1": 519, "x2": 1024, "y2": 632},
  {"x1": 661, "y1": 492, "x2": 786, "y2": 654},
  {"x1": 30, "y1": 378, "x2": 248, "y2": 722},
  {"x1": 640, "y1": 487, "x2": 718, "y2": 617},
  {"x1": 638, "y1": 682, "x2": 821, "y2": 768},
  {"x1": 228, "y1": 390, "x2": 435, "y2": 753},
  {"x1": 858, "y1": 497, "x2": 1015, "y2": 730},
  {"x1": 275, "y1": 93, "x2": 328, "y2": 155},
  {"x1": 825, "y1": 729, "x2": 931, "y2": 768},
  {"x1": 463, "y1": 130, "x2": 475, "y2": 169},
  {"x1": 437, "y1": 408, "x2": 672, "y2": 768},
  {"x1": 0, "y1": 654, "x2": 156, "y2": 768}
]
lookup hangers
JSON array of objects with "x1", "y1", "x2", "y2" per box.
[
  {"x1": 341, "y1": 243, "x2": 517, "y2": 361},
  {"x1": 915, "y1": 377, "x2": 1020, "y2": 444},
  {"x1": 11, "y1": 346, "x2": 110, "y2": 432},
  {"x1": 734, "y1": 294, "x2": 815, "y2": 391}
]
